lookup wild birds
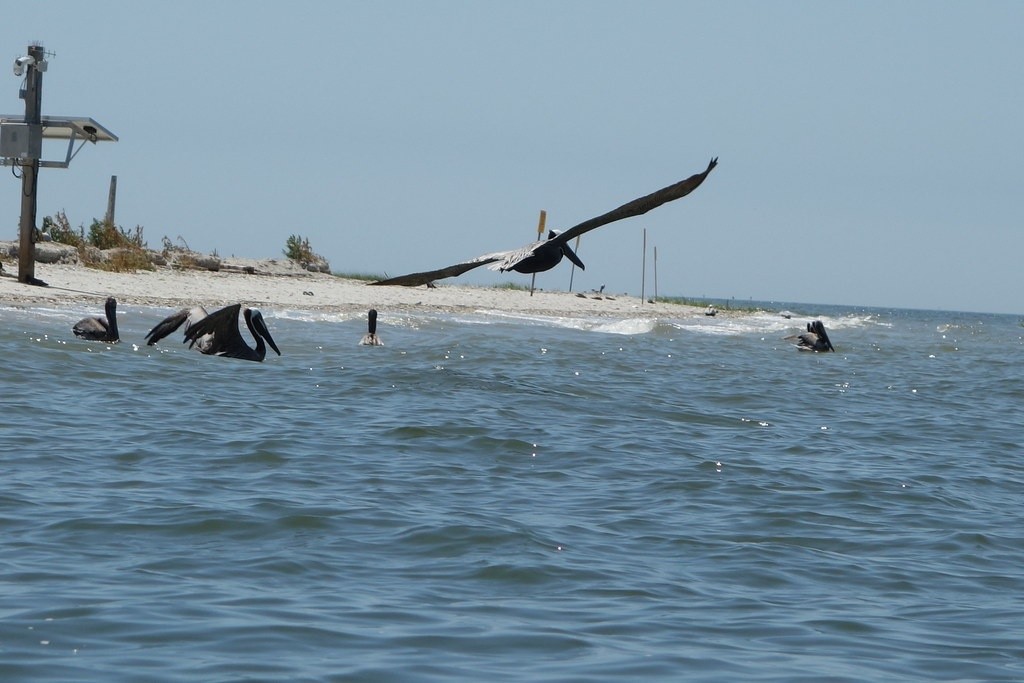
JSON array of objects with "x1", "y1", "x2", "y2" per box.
[
  {"x1": 357, "y1": 307, "x2": 385, "y2": 347},
  {"x1": 780, "y1": 319, "x2": 835, "y2": 354},
  {"x1": 143, "y1": 303, "x2": 281, "y2": 362},
  {"x1": 703, "y1": 304, "x2": 717, "y2": 317},
  {"x1": 71, "y1": 296, "x2": 121, "y2": 345},
  {"x1": 364, "y1": 149, "x2": 719, "y2": 288}
]
